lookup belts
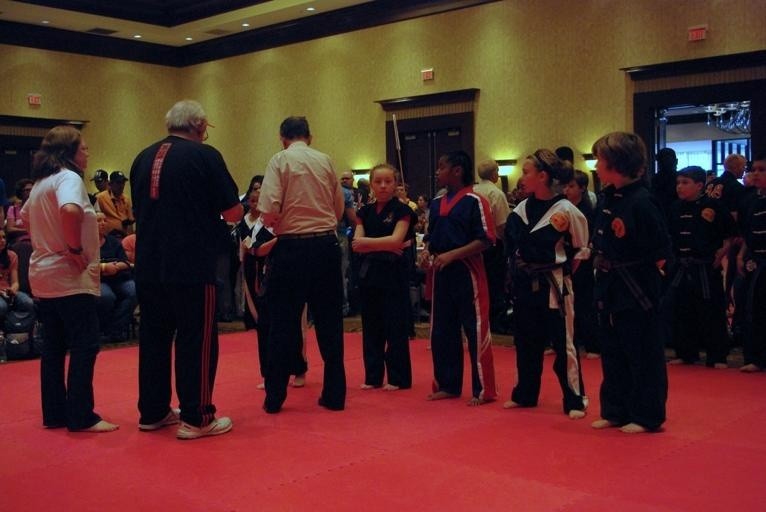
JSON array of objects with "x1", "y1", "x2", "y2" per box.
[{"x1": 277, "y1": 230, "x2": 335, "y2": 239}]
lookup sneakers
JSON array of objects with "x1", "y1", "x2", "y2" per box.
[
  {"x1": 176, "y1": 416, "x2": 232, "y2": 438},
  {"x1": 138, "y1": 408, "x2": 182, "y2": 430}
]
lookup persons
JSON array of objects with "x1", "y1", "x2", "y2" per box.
[
  {"x1": 418, "y1": 147, "x2": 497, "y2": 406},
  {"x1": 1, "y1": 170, "x2": 135, "y2": 361},
  {"x1": 588, "y1": 132, "x2": 670, "y2": 432},
  {"x1": 20, "y1": 126, "x2": 119, "y2": 432},
  {"x1": 214, "y1": 174, "x2": 308, "y2": 389},
  {"x1": 348, "y1": 163, "x2": 418, "y2": 388},
  {"x1": 732, "y1": 154, "x2": 764, "y2": 369},
  {"x1": 337, "y1": 171, "x2": 432, "y2": 321},
  {"x1": 472, "y1": 145, "x2": 602, "y2": 359},
  {"x1": 128, "y1": 102, "x2": 244, "y2": 439},
  {"x1": 652, "y1": 148, "x2": 746, "y2": 368},
  {"x1": 501, "y1": 148, "x2": 587, "y2": 418},
  {"x1": 255, "y1": 116, "x2": 347, "y2": 412}
]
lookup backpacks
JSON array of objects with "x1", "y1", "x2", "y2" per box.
[{"x1": 3, "y1": 311, "x2": 42, "y2": 360}]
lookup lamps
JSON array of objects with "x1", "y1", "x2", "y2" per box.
[
  {"x1": 583, "y1": 153, "x2": 601, "y2": 196},
  {"x1": 352, "y1": 169, "x2": 371, "y2": 183},
  {"x1": 494, "y1": 159, "x2": 517, "y2": 193},
  {"x1": 705, "y1": 101, "x2": 751, "y2": 135}
]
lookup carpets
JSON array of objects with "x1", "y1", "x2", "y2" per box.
[{"x1": 1, "y1": 325, "x2": 766, "y2": 511}]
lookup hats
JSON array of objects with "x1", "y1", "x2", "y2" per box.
[
  {"x1": 110, "y1": 171, "x2": 128, "y2": 181},
  {"x1": 90, "y1": 169, "x2": 108, "y2": 180}
]
